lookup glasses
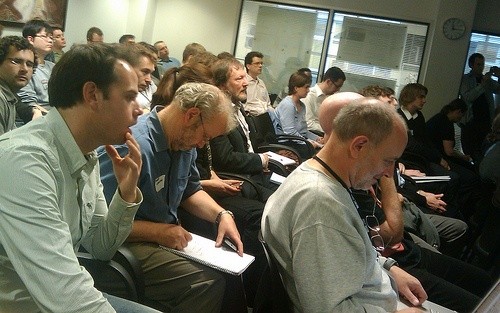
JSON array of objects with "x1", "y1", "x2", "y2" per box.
[
  {"x1": 365, "y1": 178, "x2": 392, "y2": 253},
  {"x1": 5, "y1": 54, "x2": 37, "y2": 69},
  {"x1": 331, "y1": 80, "x2": 343, "y2": 88},
  {"x1": 34, "y1": 31, "x2": 53, "y2": 41},
  {"x1": 251, "y1": 62, "x2": 264, "y2": 65},
  {"x1": 200, "y1": 114, "x2": 215, "y2": 142}
]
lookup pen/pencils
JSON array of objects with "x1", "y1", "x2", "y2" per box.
[{"x1": 176, "y1": 219, "x2": 188, "y2": 248}]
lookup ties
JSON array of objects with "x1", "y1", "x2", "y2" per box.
[{"x1": 169, "y1": 150, "x2": 178, "y2": 220}]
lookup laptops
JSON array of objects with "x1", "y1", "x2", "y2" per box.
[{"x1": 396, "y1": 280, "x2": 500, "y2": 313}]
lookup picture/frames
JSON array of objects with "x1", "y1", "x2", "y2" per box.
[{"x1": 0, "y1": 0, "x2": 69, "y2": 33}]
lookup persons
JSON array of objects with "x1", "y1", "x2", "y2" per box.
[
  {"x1": 16, "y1": 19, "x2": 57, "y2": 114},
  {"x1": 459, "y1": 53, "x2": 500, "y2": 174},
  {"x1": 44, "y1": 23, "x2": 66, "y2": 64},
  {"x1": 0, "y1": 35, "x2": 47, "y2": 136},
  {"x1": 0, "y1": 43, "x2": 165, "y2": 313},
  {"x1": 150, "y1": 52, "x2": 346, "y2": 313},
  {"x1": 78, "y1": 81, "x2": 243, "y2": 313},
  {"x1": 87, "y1": 27, "x2": 104, "y2": 44},
  {"x1": 119, "y1": 35, "x2": 207, "y2": 120},
  {"x1": 261, "y1": 98, "x2": 428, "y2": 313},
  {"x1": 319, "y1": 83, "x2": 500, "y2": 313}
]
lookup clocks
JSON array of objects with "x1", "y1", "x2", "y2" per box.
[{"x1": 443, "y1": 18, "x2": 466, "y2": 40}]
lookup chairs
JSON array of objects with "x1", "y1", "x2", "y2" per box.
[{"x1": 72, "y1": 112, "x2": 500, "y2": 313}]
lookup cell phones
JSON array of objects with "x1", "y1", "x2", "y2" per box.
[{"x1": 222, "y1": 235, "x2": 238, "y2": 252}]
